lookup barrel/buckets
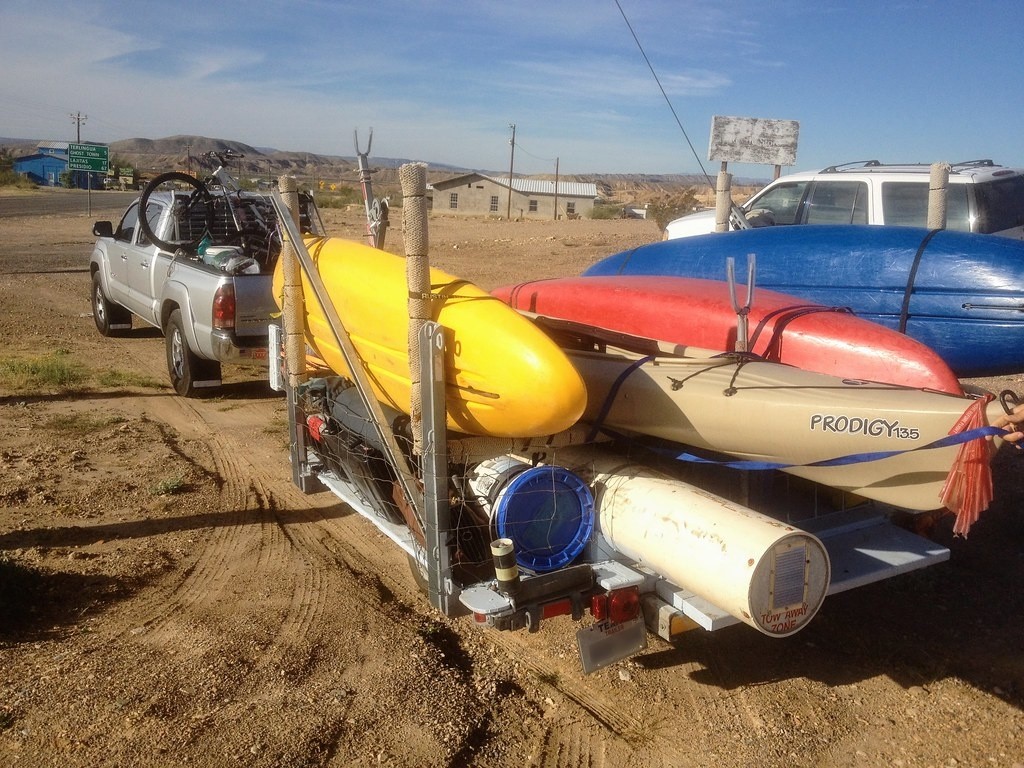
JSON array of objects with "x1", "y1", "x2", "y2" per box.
[
  {"x1": 203, "y1": 245, "x2": 242, "y2": 276},
  {"x1": 465, "y1": 455, "x2": 597, "y2": 572}
]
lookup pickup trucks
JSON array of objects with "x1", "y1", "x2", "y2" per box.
[{"x1": 89, "y1": 187, "x2": 341, "y2": 400}]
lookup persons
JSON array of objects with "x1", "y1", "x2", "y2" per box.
[{"x1": 985, "y1": 404, "x2": 1024, "y2": 449}]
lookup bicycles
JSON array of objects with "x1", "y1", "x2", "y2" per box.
[{"x1": 137, "y1": 146, "x2": 282, "y2": 272}]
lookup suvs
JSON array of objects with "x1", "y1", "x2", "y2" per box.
[{"x1": 659, "y1": 159, "x2": 1024, "y2": 258}]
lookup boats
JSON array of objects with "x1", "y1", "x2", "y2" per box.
[
  {"x1": 489, "y1": 275, "x2": 963, "y2": 400},
  {"x1": 271, "y1": 230, "x2": 589, "y2": 440},
  {"x1": 548, "y1": 323, "x2": 1023, "y2": 516},
  {"x1": 582, "y1": 224, "x2": 1024, "y2": 376}
]
